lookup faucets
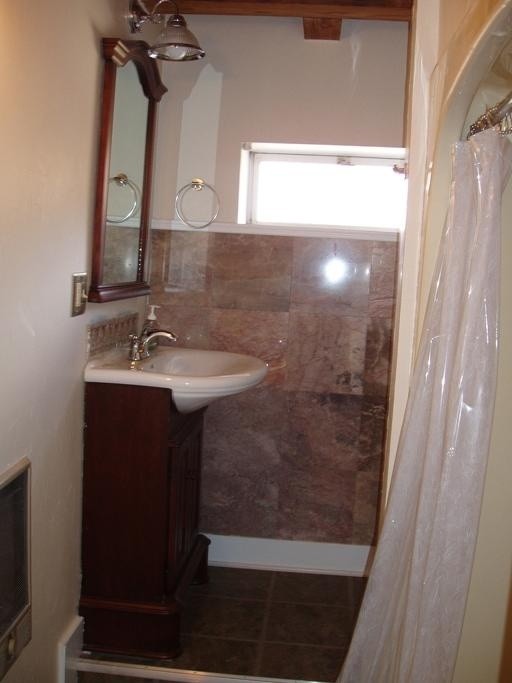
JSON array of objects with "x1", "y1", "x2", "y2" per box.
[{"x1": 127, "y1": 330, "x2": 179, "y2": 361}]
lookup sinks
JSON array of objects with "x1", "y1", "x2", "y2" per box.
[{"x1": 133, "y1": 347, "x2": 268, "y2": 380}]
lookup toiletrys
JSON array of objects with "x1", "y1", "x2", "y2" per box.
[{"x1": 143, "y1": 304, "x2": 162, "y2": 351}]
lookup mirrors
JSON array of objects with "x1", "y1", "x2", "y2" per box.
[{"x1": 88, "y1": 38, "x2": 168, "y2": 303}]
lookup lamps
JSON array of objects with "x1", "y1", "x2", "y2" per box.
[{"x1": 128, "y1": 0, "x2": 204, "y2": 62}]
